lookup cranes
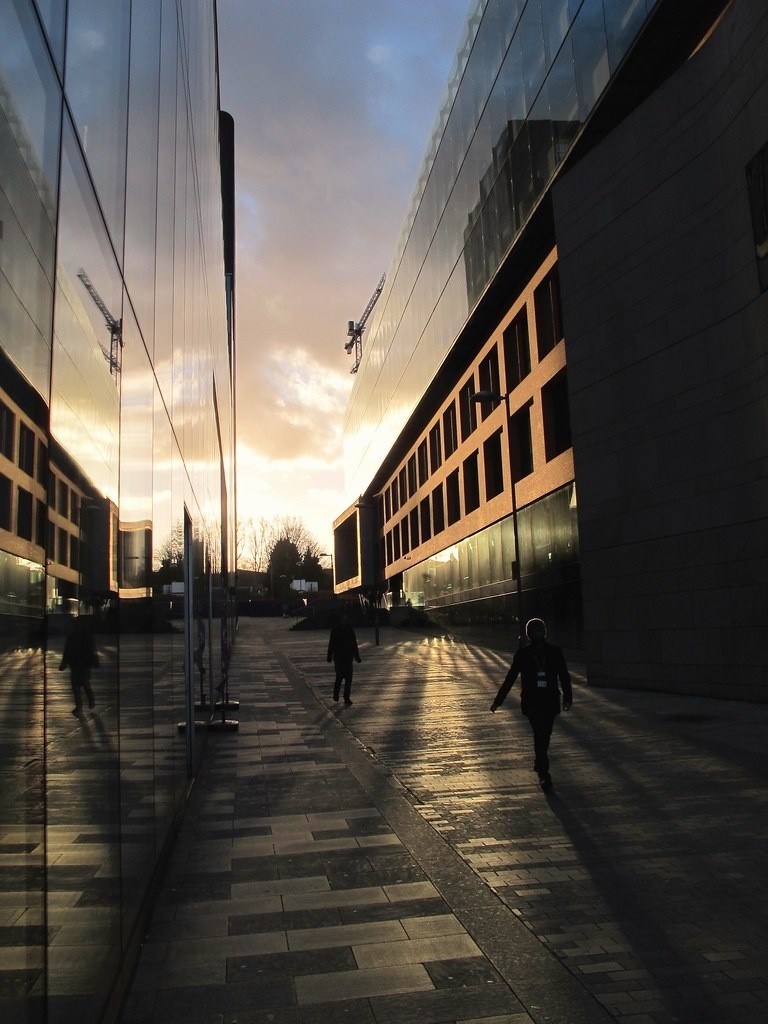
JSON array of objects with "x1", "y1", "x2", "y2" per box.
[{"x1": 344, "y1": 271, "x2": 386, "y2": 376}]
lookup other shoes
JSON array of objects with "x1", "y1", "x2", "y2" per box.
[
  {"x1": 539, "y1": 768, "x2": 552, "y2": 791},
  {"x1": 533, "y1": 759, "x2": 541, "y2": 772}
]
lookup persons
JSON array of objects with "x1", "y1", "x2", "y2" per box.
[
  {"x1": 60, "y1": 615, "x2": 101, "y2": 712},
  {"x1": 327, "y1": 610, "x2": 361, "y2": 704},
  {"x1": 490, "y1": 619, "x2": 572, "y2": 787}
]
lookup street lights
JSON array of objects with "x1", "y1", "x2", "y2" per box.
[
  {"x1": 280, "y1": 575, "x2": 288, "y2": 603},
  {"x1": 319, "y1": 552, "x2": 334, "y2": 593},
  {"x1": 472, "y1": 390, "x2": 526, "y2": 645},
  {"x1": 355, "y1": 492, "x2": 382, "y2": 646},
  {"x1": 296, "y1": 561, "x2": 304, "y2": 593}
]
